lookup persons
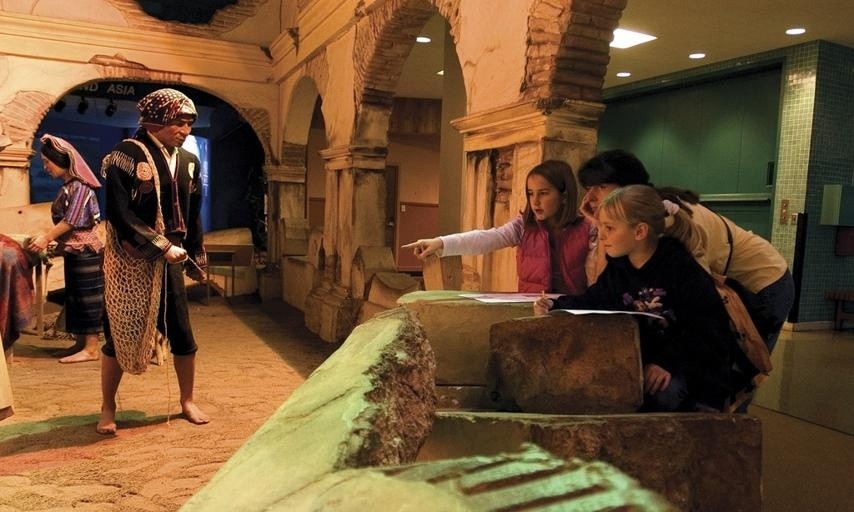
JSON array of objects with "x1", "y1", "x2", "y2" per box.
[
  {"x1": 96, "y1": 87, "x2": 213, "y2": 434},
  {"x1": 400, "y1": 159, "x2": 596, "y2": 296},
  {"x1": 28, "y1": 133, "x2": 106, "y2": 363},
  {"x1": 579, "y1": 148, "x2": 795, "y2": 414},
  {"x1": 0, "y1": 234, "x2": 36, "y2": 420},
  {"x1": 533, "y1": 184, "x2": 741, "y2": 412}
]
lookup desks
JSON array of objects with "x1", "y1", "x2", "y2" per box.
[{"x1": 206, "y1": 250, "x2": 235, "y2": 304}]
